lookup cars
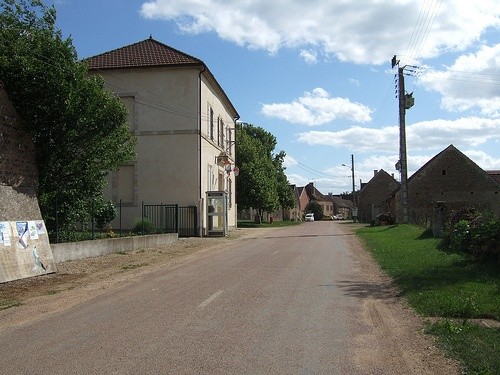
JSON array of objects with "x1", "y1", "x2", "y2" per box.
[
  {"x1": 305, "y1": 214, "x2": 314, "y2": 221},
  {"x1": 331, "y1": 213, "x2": 343, "y2": 220}
]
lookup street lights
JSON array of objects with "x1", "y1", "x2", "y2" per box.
[{"x1": 342, "y1": 164, "x2": 356, "y2": 209}]
